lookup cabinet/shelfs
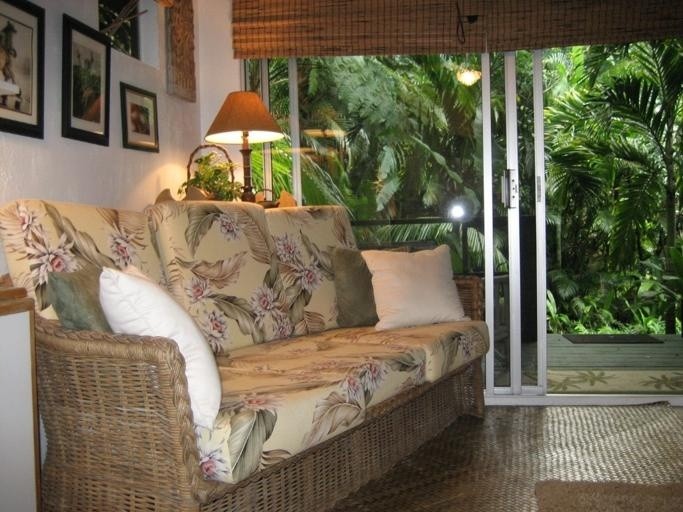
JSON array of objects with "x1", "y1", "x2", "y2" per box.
[{"x1": 458, "y1": 272, "x2": 509, "y2": 368}]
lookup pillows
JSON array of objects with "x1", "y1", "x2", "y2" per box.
[
  {"x1": 326, "y1": 243, "x2": 408, "y2": 328},
  {"x1": 99, "y1": 263, "x2": 221, "y2": 430},
  {"x1": 46, "y1": 265, "x2": 111, "y2": 334},
  {"x1": 360, "y1": 244, "x2": 471, "y2": 330}
]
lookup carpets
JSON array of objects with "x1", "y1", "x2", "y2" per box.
[
  {"x1": 562, "y1": 334, "x2": 665, "y2": 344},
  {"x1": 534, "y1": 479, "x2": 682, "y2": 512},
  {"x1": 522, "y1": 365, "x2": 682, "y2": 395}
]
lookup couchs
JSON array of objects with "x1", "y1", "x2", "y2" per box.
[{"x1": 0, "y1": 198, "x2": 488, "y2": 512}]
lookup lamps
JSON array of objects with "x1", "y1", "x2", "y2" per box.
[{"x1": 205, "y1": 92, "x2": 284, "y2": 202}]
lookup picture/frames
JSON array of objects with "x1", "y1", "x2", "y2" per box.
[
  {"x1": 0, "y1": 0, "x2": 44, "y2": 139},
  {"x1": 120, "y1": 81, "x2": 159, "y2": 151},
  {"x1": 62, "y1": 13, "x2": 111, "y2": 147},
  {"x1": 158, "y1": 0, "x2": 198, "y2": 103}
]
